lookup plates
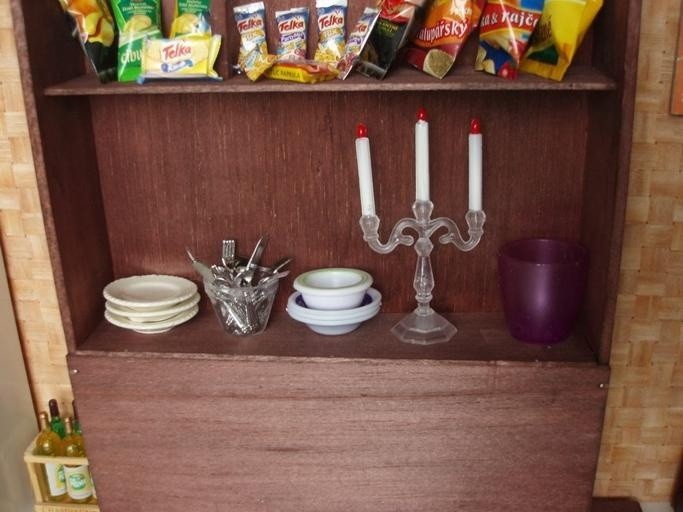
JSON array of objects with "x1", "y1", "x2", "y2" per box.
[{"x1": 102, "y1": 274, "x2": 201, "y2": 335}]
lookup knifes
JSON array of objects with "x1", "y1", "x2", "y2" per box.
[{"x1": 242, "y1": 235, "x2": 267, "y2": 284}]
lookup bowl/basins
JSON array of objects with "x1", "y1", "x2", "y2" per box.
[{"x1": 285, "y1": 267, "x2": 383, "y2": 336}]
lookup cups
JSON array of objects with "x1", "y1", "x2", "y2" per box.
[
  {"x1": 495, "y1": 238, "x2": 590, "y2": 344},
  {"x1": 203, "y1": 265, "x2": 278, "y2": 338}
]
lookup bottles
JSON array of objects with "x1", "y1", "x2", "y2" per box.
[{"x1": 33, "y1": 399, "x2": 97, "y2": 505}]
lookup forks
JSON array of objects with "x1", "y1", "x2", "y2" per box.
[{"x1": 222, "y1": 238, "x2": 236, "y2": 279}]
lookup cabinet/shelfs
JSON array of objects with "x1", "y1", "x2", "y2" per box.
[{"x1": 10, "y1": 0, "x2": 642, "y2": 512}]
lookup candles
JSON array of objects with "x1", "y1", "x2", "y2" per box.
[
  {"x1": 415, "y1": 104, "x2": 430, "y2": 202},
  {"x1": 355, "y1": 122, "x2": 376, "y2": 216},
  {"x1": 469, "y1": 119, "x2": 483, "y2": 212}
]
